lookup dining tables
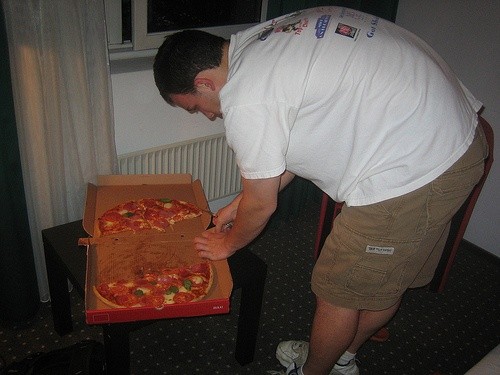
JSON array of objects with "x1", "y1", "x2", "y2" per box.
[{"x1": 41, "y1": 220, "x2": 268, "y2": 375}]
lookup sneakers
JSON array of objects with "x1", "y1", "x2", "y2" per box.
[
  {"x1": 265, "y1": 361, "x2": 299, "y2": 375},
  {"x1": 277, "y1": 341, "x2": 358, "y2": 375}
]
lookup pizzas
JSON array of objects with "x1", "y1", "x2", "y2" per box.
[
  {"x1": 93, "y1": 258, "x2": 214, "y2": 308},
  {"x1": 96, "y1": 198, "x2": 203, "y2": 237}
]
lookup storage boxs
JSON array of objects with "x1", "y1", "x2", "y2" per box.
[{"x1": 79, "y1": 173, "x2": 233, "y2": 324}]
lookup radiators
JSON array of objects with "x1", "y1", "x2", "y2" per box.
[{"x1": 116, "y1": 132, "x2": 243, "y2": 202}]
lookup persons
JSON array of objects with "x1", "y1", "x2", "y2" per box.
[{"x1": 152, "y1": 6, "x2": 490, "y2": 375}]
